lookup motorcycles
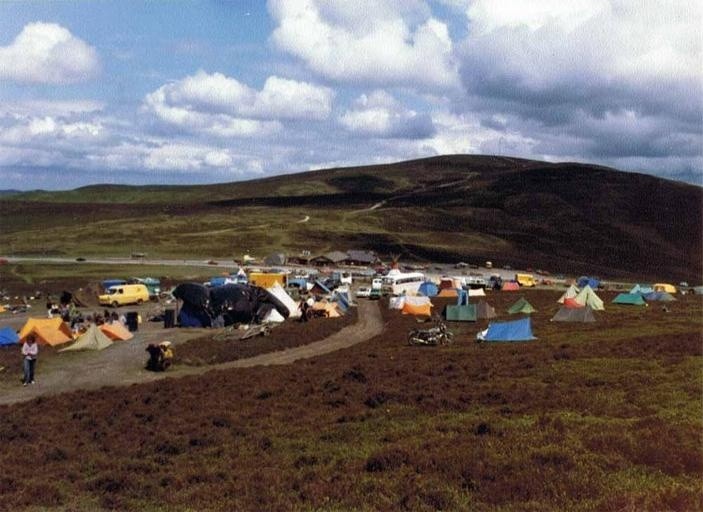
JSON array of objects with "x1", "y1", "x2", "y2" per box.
[{"x1": 407, "y1": 320, "x2": 455, "y2": 344}]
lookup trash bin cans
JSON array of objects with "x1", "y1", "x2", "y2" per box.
[
  {"x1": 127, "y1": 312, "x2": 139, "y2": 331},
  {"x1": 164, "y1": 309, "x2": 175, "y2": 328}
]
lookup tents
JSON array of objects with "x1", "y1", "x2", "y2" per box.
[
  {"x1": 556, "y1": 284, "x2": 580, "y2": 305},
  {"x1": 629, "y1": 283, "x2": 652, "y2": 295},
  {"x1": 499, "y1": 282, "x2": 519, "y2": 291},
  {"x1": 475, "y1": 317, "x2": 535, "y2": 342},
  {"x1": 548, "y1": 303, "x2": 596, "y2": 323},
  {"x1": 388, "y1": 279, "x2": 496, "y2": 326},
  {"x1": 643, "y1": 289, "x2": 678, "y2": 302},
  {"x1": 609, "y1": 292, "x2": 644, "y2": 307},
  {"x1": 505, "y1": 296, "x2": 537, "y2": 315},
  {"x1": 0, "y1": 315, "x2": 133, "y2": 354},
  {"x1": 574, "y1": 284, "x2": 605, "y2": 311}
]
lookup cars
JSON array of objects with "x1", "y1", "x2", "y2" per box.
[{"x1": 355, "y1": 278, "x2": 381, "y2": 299}]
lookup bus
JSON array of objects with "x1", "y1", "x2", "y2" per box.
[{"x1": 380, "y1": 272, "x2": 425, "y2": 296}]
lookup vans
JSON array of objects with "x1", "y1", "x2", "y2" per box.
[
  {"x1": 472, "y1": 279, "x2": 486, "y2": 288},
  {"x1": 98, "y1": 284, "x2": 149, "y2": 306},
  {"x1": 516, "y1": 274, "x2": 536, "y2": 287}
]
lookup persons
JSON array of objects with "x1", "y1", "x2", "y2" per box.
[
  {"x1": 19, "y1": 335, "x2": 36, "y2": 386},
  {"x1": 154, "y1": 343, "x2": 172, "y2": 370},
  {"x1": 11, "y1": 295, "x2": 118, "y2": 332}
]
